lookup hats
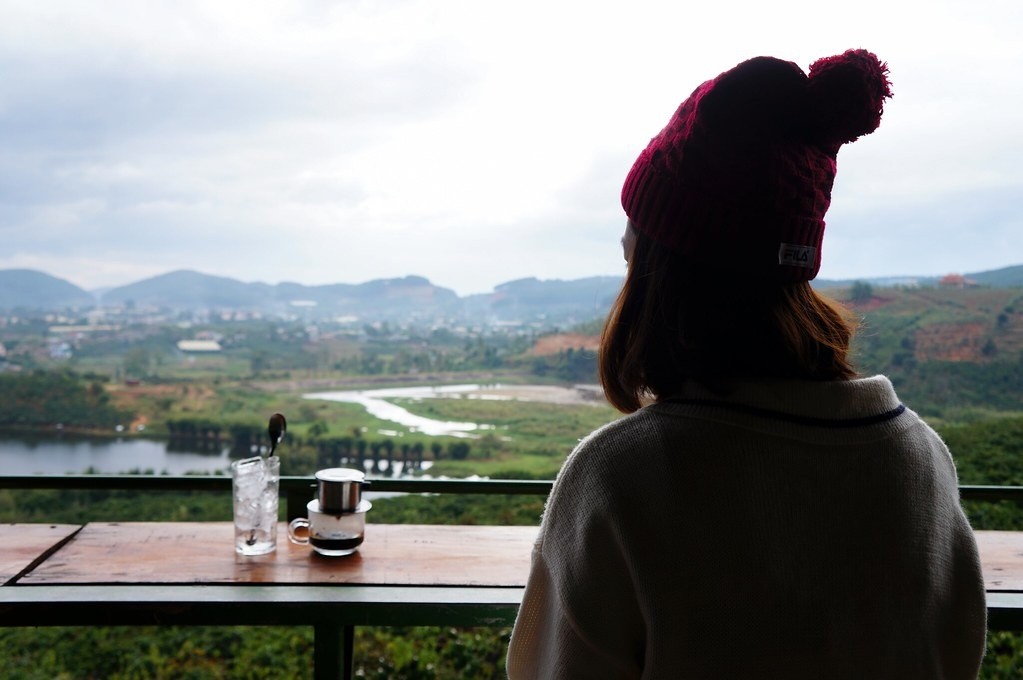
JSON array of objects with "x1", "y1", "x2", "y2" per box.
[{"x1": 622, "y1": 48, "x2": 893, "y2": 279}]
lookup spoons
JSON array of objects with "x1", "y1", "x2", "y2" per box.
[{"x1": 246, "y1": 412, "x2": 287, "y2": 545}]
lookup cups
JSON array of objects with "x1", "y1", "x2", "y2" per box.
[
  {"x1": 315, "y1": 468, "x2": 365, "y2": 513},
  {"x1": 287, "y1": 499, "x2": 373, "y2": 558},
  {"x1": 231, "y1": 456, "x2": 281, "y2": 555}
]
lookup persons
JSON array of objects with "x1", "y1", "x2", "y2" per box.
[{"x1": 505, "y1": 48, "x2": 990, "y2": 680}]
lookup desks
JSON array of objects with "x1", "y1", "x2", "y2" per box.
[{"x1": 0, "y1": 522, "x2": 1023, "y2": 679}]
